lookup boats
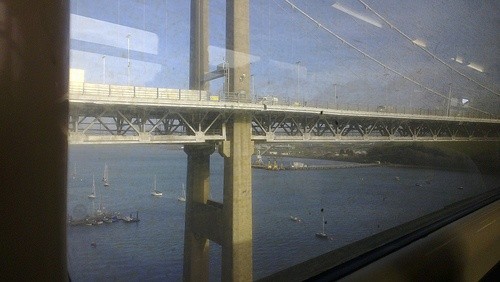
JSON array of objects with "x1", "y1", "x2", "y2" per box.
[{"x1": 290, "y1": 172, "x2": 425, "y2": 240}]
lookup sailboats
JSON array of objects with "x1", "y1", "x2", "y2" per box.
[{"x1": 72, "y1": 161, "x2": 187, "y2": 253}]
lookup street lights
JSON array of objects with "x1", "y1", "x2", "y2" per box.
[
  {"x1": 126, "y1": 34, "x2": 131, "y2": 85},
  {"x1": 296, "y1": 61, "x2": 301, "y2": 102},
  {"x1": 334, "y1": 82, "x2": 336, "y2": 110},
  {"x1": 102, "y1": 55, "x2": 106, "y2": 85}
]
